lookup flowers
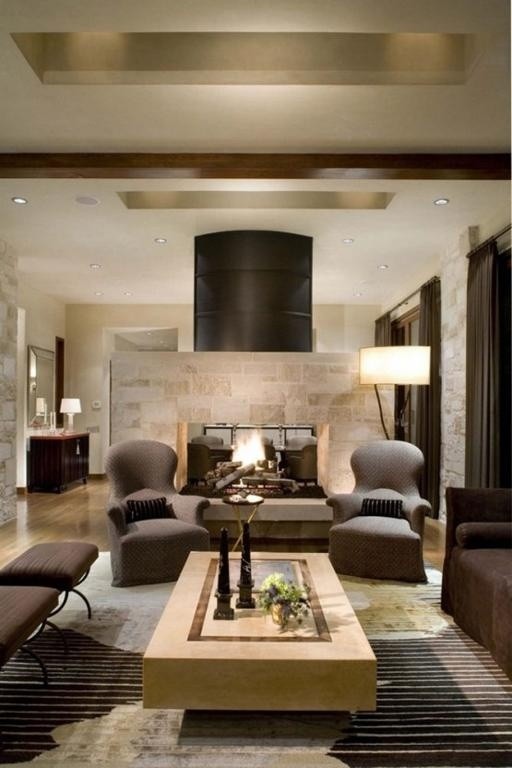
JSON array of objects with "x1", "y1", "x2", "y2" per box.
[{"x1": 252, "y1": 570, "x2": 315, "y2": 633}]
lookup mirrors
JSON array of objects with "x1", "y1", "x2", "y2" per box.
[{"x1": 26, "y1": 344, "x2": 58, "y2": 430}]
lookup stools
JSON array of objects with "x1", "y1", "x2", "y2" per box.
[
  {"x1": 0, "y1": 540, "x2": 100, "y2": 654},
  {"x1": 0, "y1": 585, "x2": 64, "y2": 687}
]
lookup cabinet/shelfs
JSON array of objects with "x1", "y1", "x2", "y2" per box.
[{"x1": 25, "y1": 429, "x2": 91, "y2": 494}]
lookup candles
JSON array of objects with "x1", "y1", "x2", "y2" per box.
[
  {"x1": 53, "y1": 412, "x2": 56, "y2": 425},
  {"x1": 49, "y1": 411, "x2": 52, "y2": 427},
  {"x1": 44, "y1": 403, "x2": 47, "y2": 424}
]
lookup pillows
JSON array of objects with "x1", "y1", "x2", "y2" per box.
[
  {"x1": 358, "y1": 495, "x2": 404, "y2": 519},
  {"x1": 453, "y1": 521, "x2": 511, "y2": 550},
  {"x1": 124, "y1": 497, "x2": 171, "y2": 520}
]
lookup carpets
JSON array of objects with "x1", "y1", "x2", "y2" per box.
[{"x1": 1, "y1": 544, "x2": 511, "y2": 767}]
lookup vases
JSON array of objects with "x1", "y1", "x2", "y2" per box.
[{"x1": 271, "y1": 598, "x2": 293, "y2": 628}]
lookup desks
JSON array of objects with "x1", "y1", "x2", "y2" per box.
[{"x1": 222, "y1": 493, "x2": 264, "y2": 554}]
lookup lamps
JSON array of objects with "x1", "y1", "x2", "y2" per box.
[
  {"x1": 36, "y1": 397, "x2": 49, "y2": 417},
  {"x1": 356, "y1": 344, "x2": 433, "y2": 442},
  {"x1": 59, "y1": 397, "x2": 83, "y2": 436}
]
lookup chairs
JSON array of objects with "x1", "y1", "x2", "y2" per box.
[
  {"x1": 436, "y1": 485, "x2": 511, "y2": 683},
  {"x1": 325, "y1": 439, "x2": 433, "y2": 587},
  {"x1": 185, "y1": 433, "x2": 320, "y2": 491},
  {"x1": 103, "y1": 439, "x2": 214, "y2": 589}
]
